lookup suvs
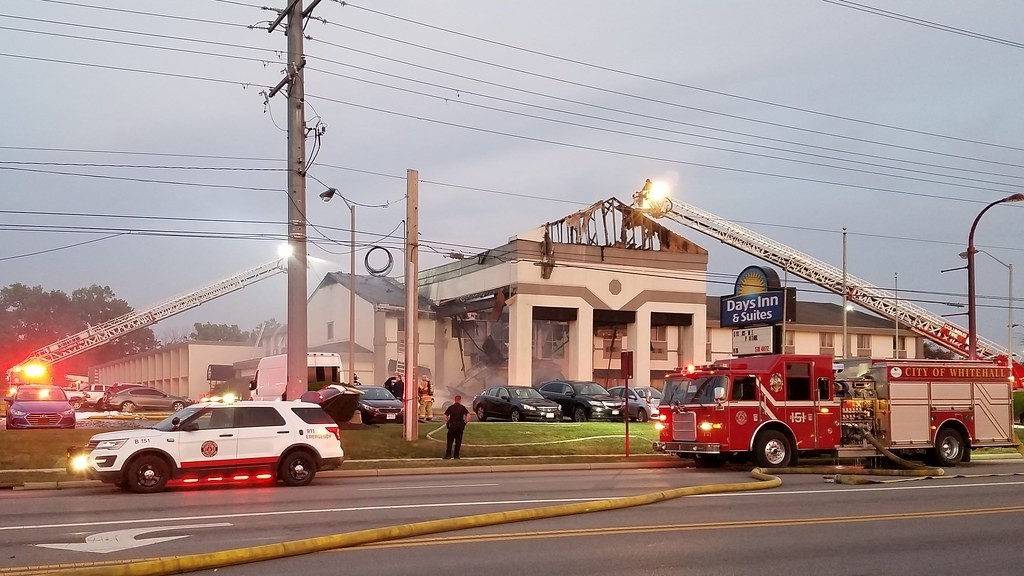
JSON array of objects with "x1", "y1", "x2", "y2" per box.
[{"x1": 83, "y1": 396, "x2": 344, "y2": 494}]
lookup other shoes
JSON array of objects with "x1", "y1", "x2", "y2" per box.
[
  {"x1": 419, "y1": 418, "x2": 425, "y2": 421},
  {"x1": 443, "y1": 457, "x2": 450, "y2": 459},
  {"x1": 426, "y1": 418, "x2": 432, "y2": 420},
  {"x1": 454, "y1": 457, "x2": 460, "y2": 459}
]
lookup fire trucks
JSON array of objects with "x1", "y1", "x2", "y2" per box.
[
  {"x1": 5, "y1": 256, "x2": 311, "y2": 397},
  {"x1": 632, "y1": 178, "x2": 1021, "y2": 468}
]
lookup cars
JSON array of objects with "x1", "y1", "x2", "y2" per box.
[
  {"x1": 4, "y1": 385, "x2": 78, "y2": 430},
  {"x1": 354, "y1": 385, "x2": 405, "y2": 425},
  {"x1": 607, "y1": 386, "x2": 662, "y2": 423},
  {"x1": 531, "y1": 380, "x2": 630, "y2": 423},
  {"x1": 472, "y1": 386, "x2": 563, "y2": 422},
  {"x1": 103, "y1": 383, "x2": 148, "y2": 411},
  {"x1": 106, "y1": 387, "x2": 192, "y2": 412}
]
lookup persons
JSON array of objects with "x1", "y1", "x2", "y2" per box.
[
  {"x1": 391, "y1": 374, "x2": 404, "y2": 403},
  {"x1": 417, "y1": 374, "x2": 434, "y2": 420},
  {"x1": 442, "y1": 394, "x2": 472, "y2": 461},
  {"x1": 638, "y1": 178, "x2": 654, "y2": 206}
]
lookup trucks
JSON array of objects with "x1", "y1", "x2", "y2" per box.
[
  {"x1": 62, "y1": 384, "x2": 113, "y2": 412},
  {"x1": 247, "y1": 352, "x2": 344, "y2": 401}
]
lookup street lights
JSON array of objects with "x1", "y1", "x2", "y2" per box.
[
  {"x1": 958, "y1": 250, "x2": 1015, "y2": 432},
  {"x1": 319, "y1": 187, "x2": 356, "y2": 386},
  {"x1": 966, "y1": 193, "x2": 1024, "y2": 359}
]
